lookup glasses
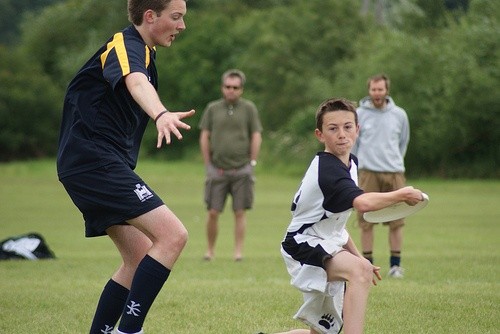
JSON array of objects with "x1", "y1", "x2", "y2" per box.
[{"x1": 224, "y1": 85, "x2": 240, "y2": 90}]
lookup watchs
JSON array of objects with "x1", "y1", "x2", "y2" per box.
[{"x1": 250, "y1": 160, "x2": 257, "y2": 168}]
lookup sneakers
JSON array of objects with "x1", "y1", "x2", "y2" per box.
[
  {"x1": 389, "y1": 267, "x2": 404, "y2": 279},
  {"x1": 115, "y1": 328, "x2": 144, "y2": 334}
]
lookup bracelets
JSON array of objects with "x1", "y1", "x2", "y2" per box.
[{"x1": 154, "y1": 110, "x2": 170, "y2": 125}]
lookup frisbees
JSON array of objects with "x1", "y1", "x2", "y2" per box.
[{"x1": 363, "y1": 193, "x2": 429, "y2": 223}]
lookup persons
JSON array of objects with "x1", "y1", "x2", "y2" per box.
[
  {"x1": 199, "y1": 70, "x2": 264, "y2": 261},
  {"x1": 254, "y1": 99, "x2": 425, "y2": 334},
  {"x1": 354, "y1": 76, "x2": 410, "y2": 280},
  {"x1": 56, "y1": 1, "x2": 196, "y2": 333}
]
